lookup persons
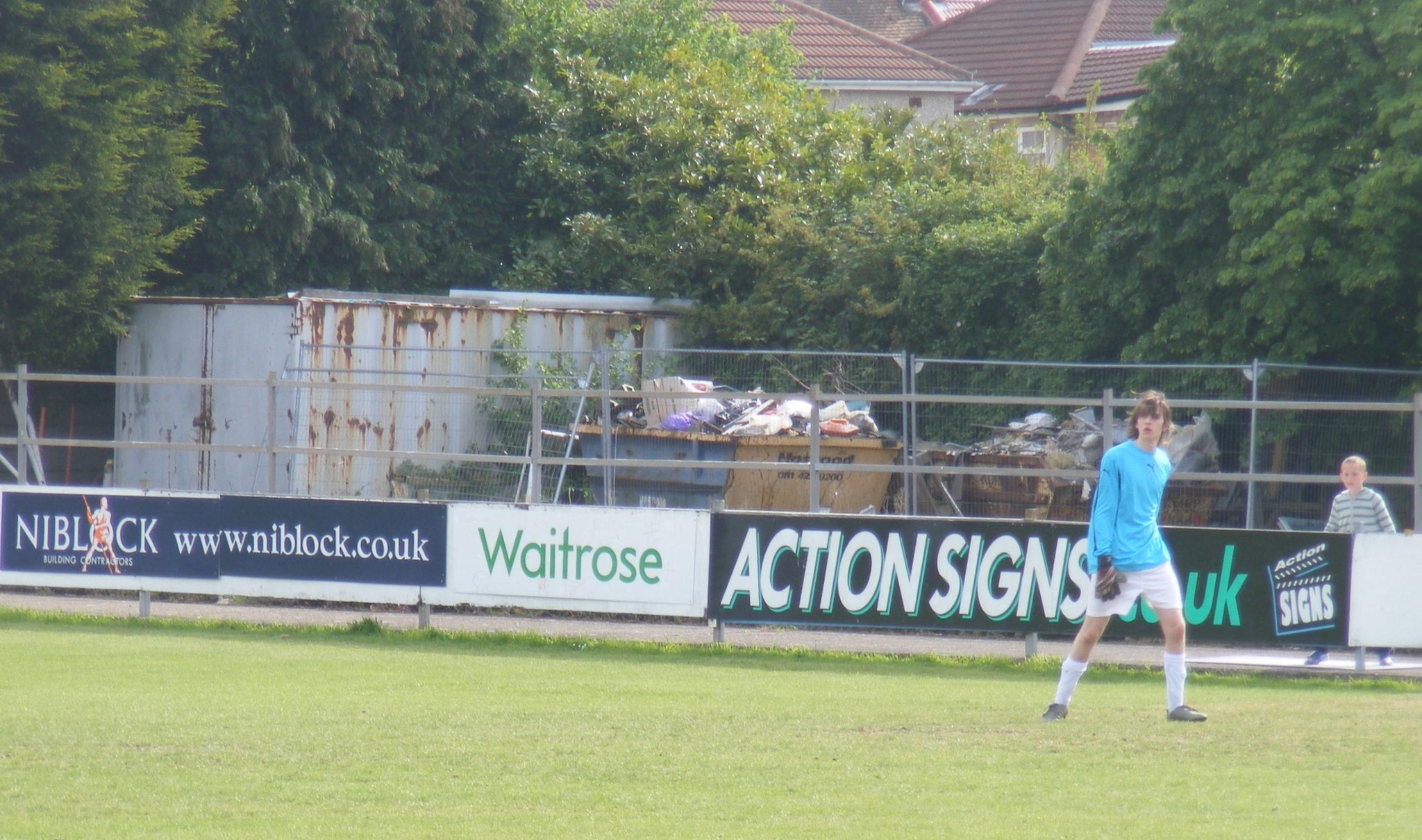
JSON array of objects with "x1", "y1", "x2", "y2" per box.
[
  {"x1": 1037, "y1": 388, "x2": 1206, "y2": 721},
  {"x1": 1301, "y1": 454, "x2": 1399, "y2": 666}
]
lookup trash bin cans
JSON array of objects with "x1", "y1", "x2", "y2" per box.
[
  {"x1": 886, "y1": 436, "x2": 1230, "y2": 528},
  {"x1": 568, "y1": 421, "x2": 903, "y2": 514}
]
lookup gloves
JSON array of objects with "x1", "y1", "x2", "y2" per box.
[{"x1": 1095, "y1": 554, "x2": 1126, "y2": 601}]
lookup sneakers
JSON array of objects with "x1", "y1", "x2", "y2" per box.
[
  {"x1": 1043, "y1": 704, "x2": 1069, "y2": 720},
  {"x1": 1304, "y1": 650, "x2": 1329, "y2": 665},
  {"x1": 1379, "y1": 652, "x2": 1392, "y2": 665},
  {"x1": 1166, "y1": 704, "x2": 1207, "y2": 722}
]
